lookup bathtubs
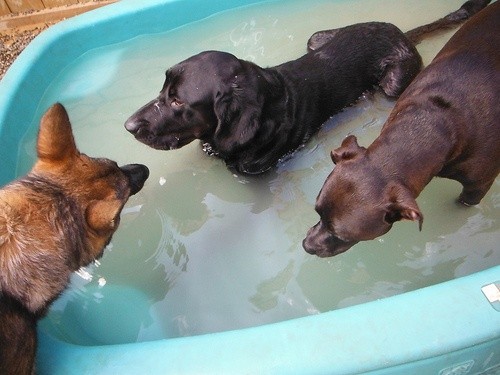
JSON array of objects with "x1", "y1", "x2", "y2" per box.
[{"x1": 1, "y1": 2, "x2": 500, "y2": 372}]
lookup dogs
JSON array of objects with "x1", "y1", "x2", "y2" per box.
[
  {"x1": 0, "y1": 103, "x2": 150, "y2": 375},
  {"x1": 123, "y1": 0, "x2": 491, "y2": 184},
  {"x1": 301, "y1": 1, "x2": 500, "y2": 259}
]
